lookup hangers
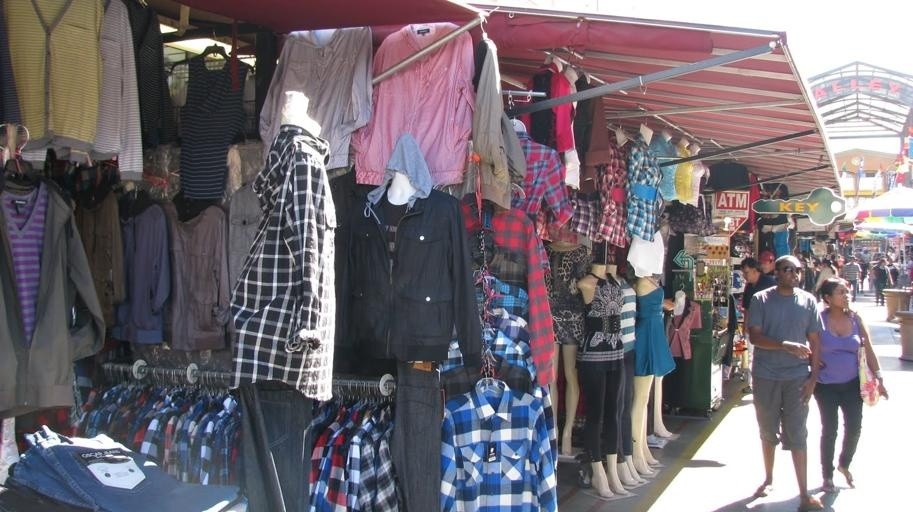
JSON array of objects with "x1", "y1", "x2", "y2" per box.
[
  {"x1": 4, "y1": 125, "x2": 32, "y2": 178},
  {"x1": 171, "y1": 27, "x2": 254, "y2": 74},
  {"x1": 475, "y1": 264, "x2": 503, "y2": 396}
]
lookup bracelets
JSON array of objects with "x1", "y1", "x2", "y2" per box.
[{"x1": 874, "y1": 370, "x2": 882, "y2": 378}]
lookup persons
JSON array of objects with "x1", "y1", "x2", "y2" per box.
[
  {"x1": 740, "y1": 257, "x2": 778, "y2": 339},
  {"x1": 797, "y1": 251, "x2": 913, "y2": 306},
  {"x1": 808, "y1": 278, "x2": 889, "y2": 494},
  {"x1": 544, "y1": 238, "x2": 673, "y2": 499},
  {"x1": 747, "y1": 254, "x2": 825, "y2": 511},
  {"x1": 664, "y1": 290, "x2": 703, "y2": 361}
]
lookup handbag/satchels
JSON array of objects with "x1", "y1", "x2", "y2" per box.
[{"x1": 857, "y1": 345, "x2": 879, "y2": 407}]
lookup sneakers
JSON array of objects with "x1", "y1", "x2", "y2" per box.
[
  {"x1": 759, "y1": 484, "x2": 773, "y2": 496},
  {"x1": 823, "y1": 477, "x2": 836, "y2": 493},
  {"x1": 837, "y1": 465, "x2": 856, "y2": 488},
  {"x1": 799, "y1": 497, "x2": 824, "y2": 512}
]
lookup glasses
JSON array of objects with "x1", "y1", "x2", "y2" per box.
[{"x1": 782, "y1": 266, "x2": 801, "y2": 274}]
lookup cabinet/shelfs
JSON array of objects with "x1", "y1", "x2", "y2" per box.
[{"x1": 663, "y1": 269, "x2": 722, "y2": 417}]
